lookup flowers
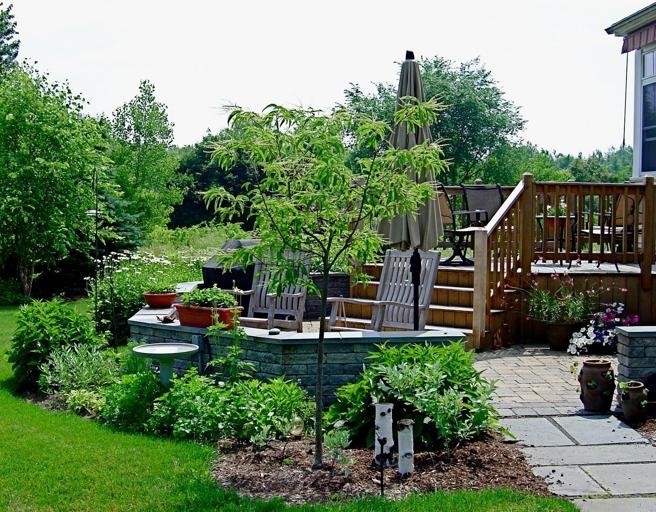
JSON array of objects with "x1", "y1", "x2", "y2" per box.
[{"x1": 502, "y1": 267, "x2": 641, "y2": 356}]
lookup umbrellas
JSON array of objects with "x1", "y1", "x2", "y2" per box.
[{"x1": 378, "y1": 48, "x2": 446, "y2": 329}]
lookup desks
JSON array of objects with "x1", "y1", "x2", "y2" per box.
[{"x1": 535, "y1": 214, "x2": 585, "y2": 270}]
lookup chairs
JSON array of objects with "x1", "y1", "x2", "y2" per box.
[
  {"x1": 581, "y1": 194, "x2": 645, "y2": 272},
  {"x1": 223, "y1": 243, "x2": 314, "y2": 332},
  {"x1": 431, "y1": 180, "x2": 514, "y2": 264},
  {"x1": 326, "y1": 248, "x2": 442, "y2": 335}
]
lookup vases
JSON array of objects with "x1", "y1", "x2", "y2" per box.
[{"x1": 578, "y1": 359, "x2": 649, "y2": 428}]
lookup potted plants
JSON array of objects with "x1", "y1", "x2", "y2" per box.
[
  {"x1": 139, "y1": 263, "x2": 177, "y2": 309},
  {"x1": 169, "y1": 285, "x2": 243, "y2": 329}
]
